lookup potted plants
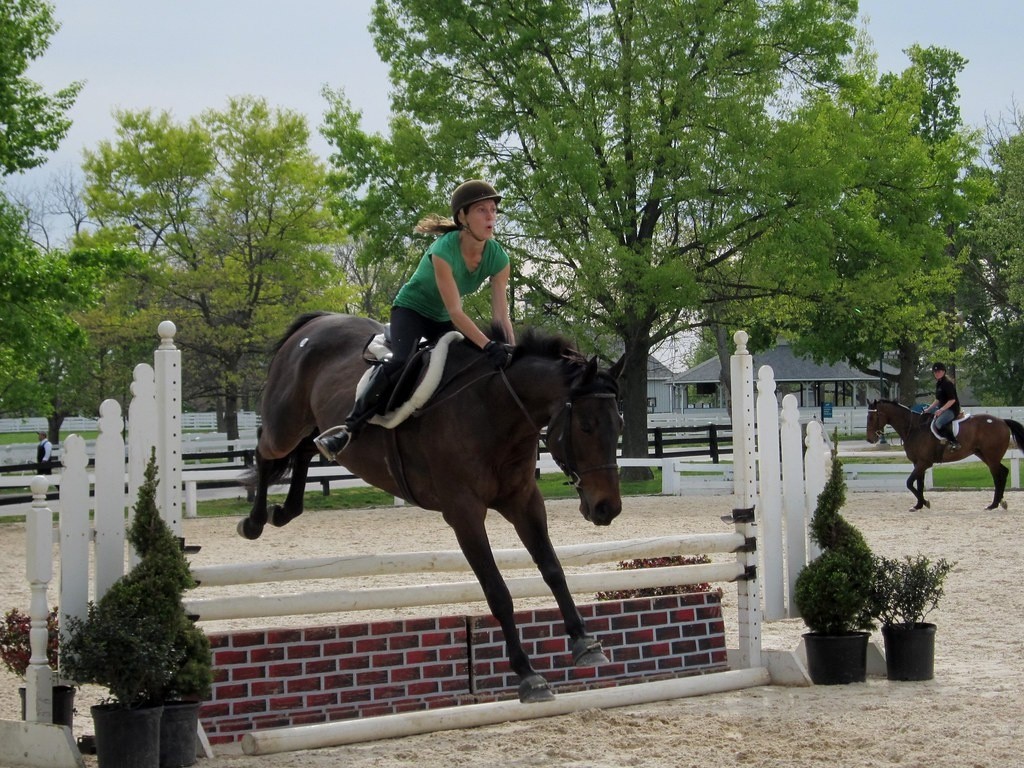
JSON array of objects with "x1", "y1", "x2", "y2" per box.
[
  {"x1": 64, "y1": 582, "x2": 176, "y2": 768},
  {"x1": 792, "y1": 550, "x2": 872, "y2": 685},
  {"x1": 0, "y1": 606, "x2": 76, "y2": 729},
  {"x1": 125, "y1": 445, "x2": 214, "y2": 768},
  {"x1": 873, "y1": 553, "x2": 959, "y2": 682}
]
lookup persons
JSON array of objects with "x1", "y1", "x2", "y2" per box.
[
  {"x1": 921, "y1": 362, "x2": 962, "y2": 454},
  {"x1": 320, "y1": 176, "x2": 516, "y2": 454},
  {"x1": 35, "y1": 431, "x2": 59, "y2": 491}
]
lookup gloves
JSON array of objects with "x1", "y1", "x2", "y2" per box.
[{"x1": 485, "y1": 339, "x2": 506, "y2": 371}]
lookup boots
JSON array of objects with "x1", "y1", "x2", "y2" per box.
[
  {"x1": 939, "y1": 425, "x2": 961, "y2": 452},
  {"x1": 318, "y1": 363, "x2": 392, "y2": 456}
]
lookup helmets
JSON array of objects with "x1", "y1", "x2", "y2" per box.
[
  {"x1": 452, "y1": 178, "x2": 502, "y2": 225},
  {"x1": 931, "y1": 363, "x2": 946, "y2": 373}
]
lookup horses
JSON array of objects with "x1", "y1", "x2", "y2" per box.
[
  {"x1": 866, "y1": 398, "x2": 1024, "y2": 512},
  {"x1": 237, "y1": 310, "x2": 624, "y2": 704}
]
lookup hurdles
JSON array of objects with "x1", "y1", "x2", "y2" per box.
[{"x1": 0, "y1": 318, "x2": 830, "y2": 768}]
